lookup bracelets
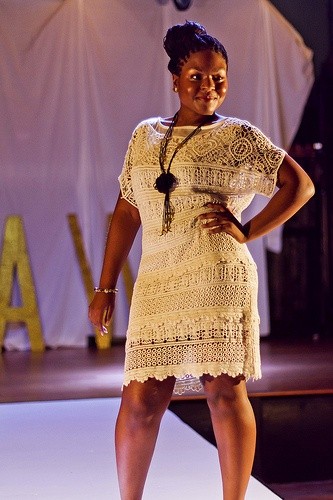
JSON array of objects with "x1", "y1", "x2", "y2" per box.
[{"x1": 92, "y1": 286, "x2": 119, "y2": 294}]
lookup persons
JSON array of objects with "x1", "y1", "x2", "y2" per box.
[{"x1": 89, "y1": 18, "x2": 314, "y2": 500}]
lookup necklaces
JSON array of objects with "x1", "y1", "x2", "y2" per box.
[{"x1": 153, "y1": 109, "x2": 210, "y2": 241}]
